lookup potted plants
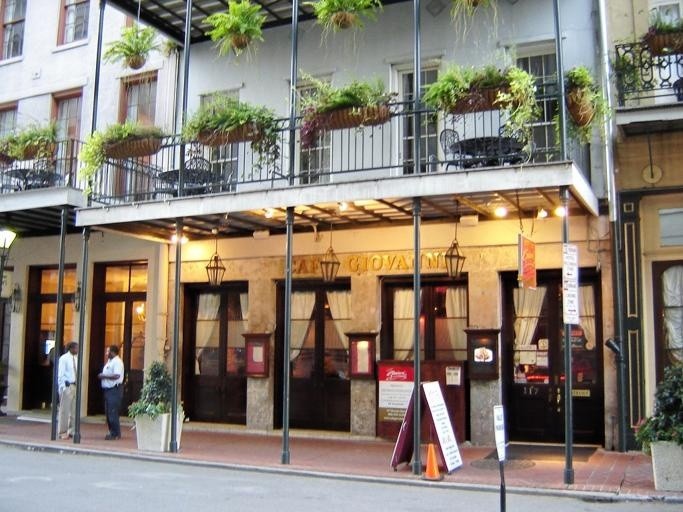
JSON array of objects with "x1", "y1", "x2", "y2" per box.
[
  {"x1": 644, "y1": 4, "x2": 683, "y2": 56},
  {"x1": 0, "y1": 115, "x2": 62, "y2": 163},
  {"x1": 419, "y1": 54, "x2": 541, "y2": 171},
  {"x1": 301, "y1": 0, "x2": 384, "y2": 56},
  {"x1": 285, "y1": 67, "x2": 400, "y2": 151},
  {"x1": 76, "y1": 116, "x2": 164, "y2": 196},
  {"x1": 177, "y1": 90, "x2": 282, "y2": 178},
  {"x1": 127, "y1": 359, "x2": 186, "y2": 452},
  {"x1": 102, "y1": 19, "x2": 164, "y2": 70},
  {"x1": 550, "y1": 66, "x2": 614, "y2": 154},
  {"x1": 199, "y1": 0, "x2": 268, "y2": 63},
  {"x1": 634, "y1": 362, "x2": 683, "y2": 492}
]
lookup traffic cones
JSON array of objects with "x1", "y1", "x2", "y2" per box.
[{"x1": 419, "y1": 442, "x2": 445, "y2": 482}]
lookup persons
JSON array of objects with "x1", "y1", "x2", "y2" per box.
[
  {"x1": 97, "y1": 344, "x2": 127, "y2": 441},
  {"x1": 55, "y1": 341, "x2": 81, "y2": 441}
]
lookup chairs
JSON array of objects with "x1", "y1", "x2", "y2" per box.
[
  {"x1": 440, "y1": 121, "x2": 528, "y2": 171},
  {"x1": 0, "y1": 157, "x2": 51, "y2": 194},
  {"x1": 145, "y1": 156, "x2": 235, "y2": 199}
]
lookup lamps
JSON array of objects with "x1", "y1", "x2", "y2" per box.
[
  {"x1": 205, "y1": 227, "x2": 226, "y2": 290},
  {"x1": 445, "y1": 199, "x2": 466, "y2": 284},
  {"x1": 320, "y1": 222, "x2": 340, "y2": 286}
]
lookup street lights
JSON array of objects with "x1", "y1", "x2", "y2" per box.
[{"x1": 0, "y1": 226, "x2": 18, "y2": 300}]
